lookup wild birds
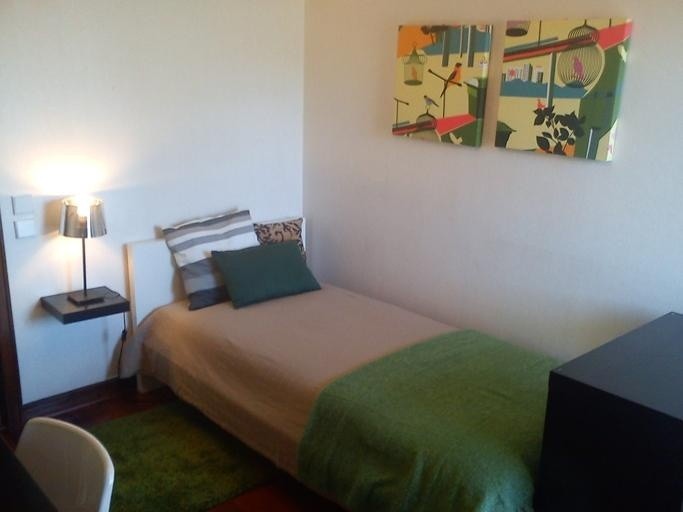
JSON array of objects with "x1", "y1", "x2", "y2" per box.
[
  {"x1": 411, "y1": 67, "x2": 419, "y2": 80},
  {"x1": 424, "y1": 95, "x2": 439, "y2": 110},
  {"x1": 439, "y1": 62, "x2": 462, "y2": 97},
  {"x1": 570, "y1": 55, "x2": 585, "y2": 79}
]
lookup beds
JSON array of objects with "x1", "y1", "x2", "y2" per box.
[{"x1": 125, "y1": 216, "x2": 566, "y2": 511}]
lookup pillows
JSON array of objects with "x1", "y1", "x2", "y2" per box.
[{"x1": 162, "y1": 209, "x2": 321, "y2": 311}]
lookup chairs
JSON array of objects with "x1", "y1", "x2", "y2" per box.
[{"x1": 13, "y1": 417, "x2": 116, "y2": 511}]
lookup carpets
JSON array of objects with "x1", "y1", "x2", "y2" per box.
[{"x1": 84, "y1": 399, "x2": 288, "y2": 512}]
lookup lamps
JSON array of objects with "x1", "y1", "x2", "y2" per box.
[{"x1": 59, "y1": 194, "x2": 107, "y2": 305}]
